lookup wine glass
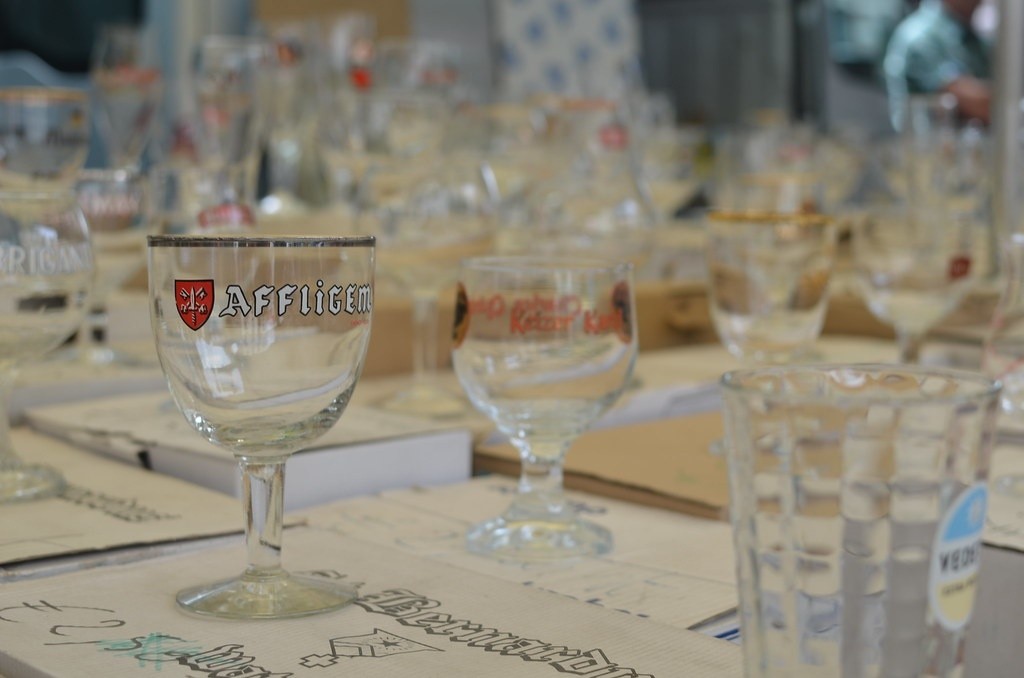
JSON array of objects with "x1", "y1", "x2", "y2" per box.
[
  {"x1": 0, "y1": 28, "x2": 1024, "y2": 504},
  {"x1": 454, "y1": 253, "x2": 638, "y2": 570},
  {"x1": 142, "y1": 228, "x2": 382, "y2": 621}
]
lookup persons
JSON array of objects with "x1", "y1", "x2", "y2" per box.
[{"x1": 884, "y1": 0, "x2": 996, "y2": 135}]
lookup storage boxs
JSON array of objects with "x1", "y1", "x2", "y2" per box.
[{"x1": 0, "y1": 244, "x2": 1024, "y2": 678}]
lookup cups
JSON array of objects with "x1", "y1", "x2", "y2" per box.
[{"x1": 716, "y1": 363, "x2": 1003, "y2": 678}]
